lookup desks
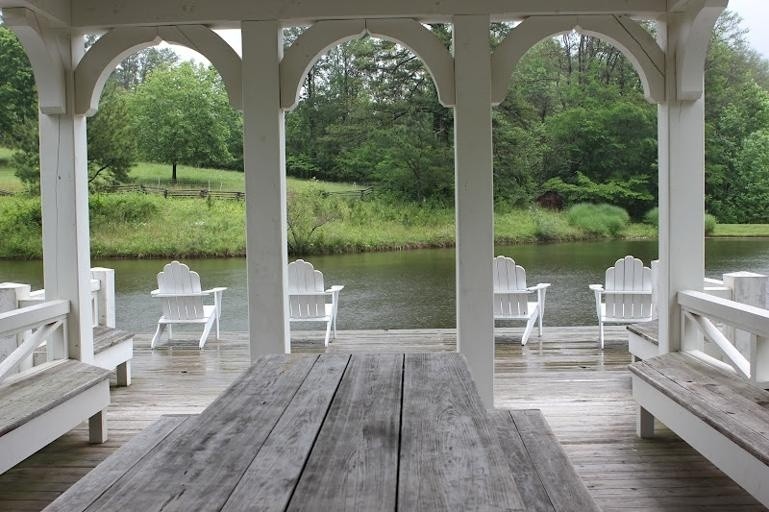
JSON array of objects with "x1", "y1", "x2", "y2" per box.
[{"x1": 101, "y1": 350, "x2": 529, "y2": 511}]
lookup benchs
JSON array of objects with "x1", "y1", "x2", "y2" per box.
[
  {"x1": 0, "y1": 358, "x2": 113, "y2": 478},
  {"x1": 38, "y1": 411, "x2": 200, "y2": 510},
  {"x1": 33, "y1": 321, "x2": 136, "y2": 386},
  {"x1": 629, "y1": 348, "x2": 769, "y2": 509},
  {"x1": 488, "y1": 407, "x2": 604, "y2": 512}
]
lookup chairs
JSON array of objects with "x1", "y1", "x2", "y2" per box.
[
  {"x1": 149, "y1": 260, "x2": 228, "y2": 349},
  {"x1": 589, "y1": 255, "x2": 654, "y2": 350},
  {"x1": 286, "y1": 259, "x2": 345, "y2": 346},
  {"x1": 493, "y1": 254, "x2": 553, "y2": 346}
]
